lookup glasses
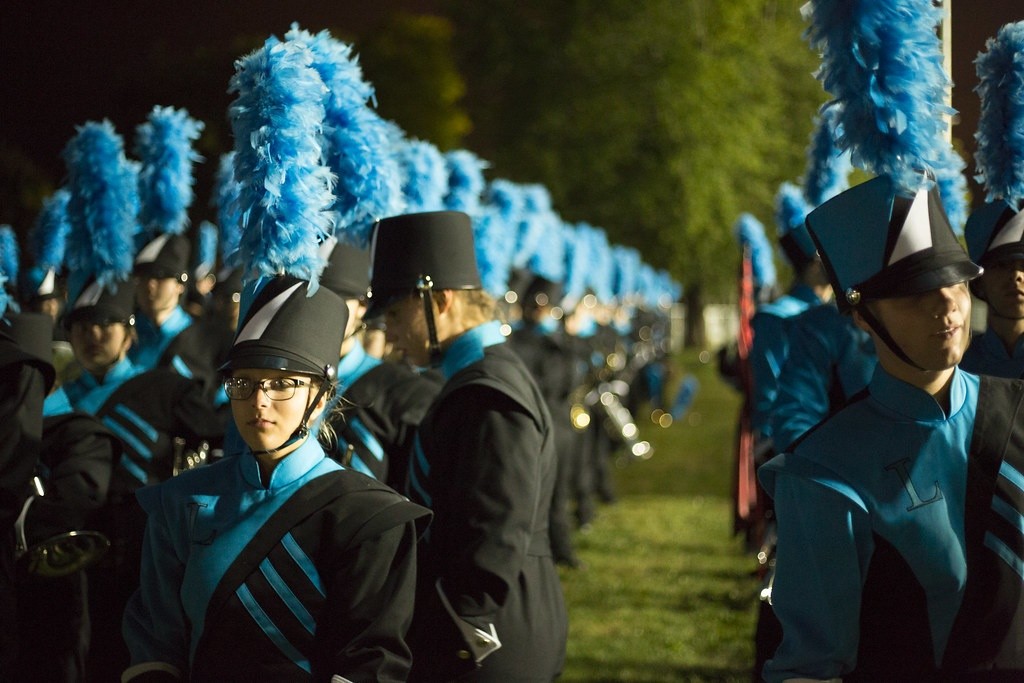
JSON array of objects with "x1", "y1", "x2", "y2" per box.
[{"x1": 224, "y1": 374, "x2": 306, "y2": 400}]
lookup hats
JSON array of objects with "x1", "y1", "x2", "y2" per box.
[
  {"x1": 734, "y1": 213, "x2": 774, "y2": 308},
  {"x1": 0, "y1": 25, "x2": 680, "y2": 375},
  {"x1": 965, "y1": 21, "x2": 1024, "y2": 298},
  {"x1": 804, "y1": 0, "x2": 985, "y2": 318},
  {"x1": 776, "y1": 183, "x2": 821, "y2": 271}
]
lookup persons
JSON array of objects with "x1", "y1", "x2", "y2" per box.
[
  {"x1": 0, "y1": 210, "x2": 668, "y2": 682},
  {"x1": 123, "y1": 275, "x2": 434, "y2": 683},
  {"x1": 715, "y1": 170, "x2": 1024, "y2": 683},
  {"x1": 966, "y1": 202, "x2": 1024, "y2": 383}
]
